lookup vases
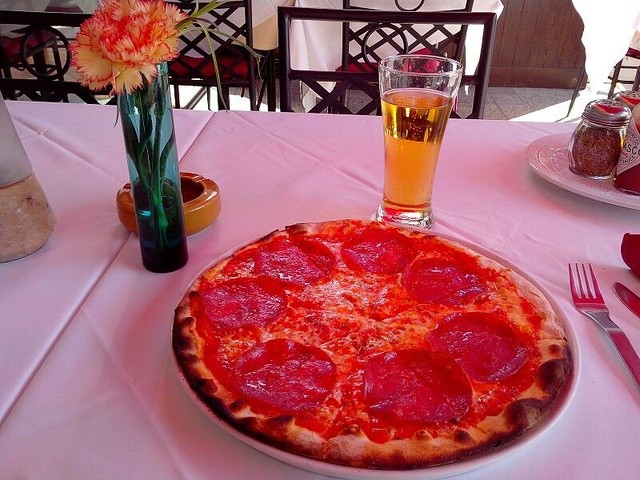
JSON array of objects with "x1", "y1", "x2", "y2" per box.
[{"x1": 121, "y1": 63, "x2": 190, "y2": 273}]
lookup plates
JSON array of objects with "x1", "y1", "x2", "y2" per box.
[{"x1": 524, "y1": 132, "x2": 640, "y2": 211}]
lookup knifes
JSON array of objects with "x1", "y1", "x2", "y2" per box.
[{"x1": 612, "y1": 280, "x2": 640, "y2": 319}]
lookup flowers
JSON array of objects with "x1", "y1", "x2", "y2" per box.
[{"x1": 65, "y1": 0, "x2": 258, "y2": 102}]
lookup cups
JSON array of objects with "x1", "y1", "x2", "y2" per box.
[{"x1": 377, "y1": 54, "x2": 465, "y2": 230}]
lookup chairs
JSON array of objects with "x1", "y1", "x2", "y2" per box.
[
  {"x1": 338, "y1": 0, "x2": 475, "y2": 119},
  {"x1": 0, "y1": 7, "x2": 119, "y2": 106},
  {"x1": 150, "y1": 1, "x2": 257, "y2": 111},
  {"x1": 277, "y1": 6, "x2": 496, "y2": 120}
]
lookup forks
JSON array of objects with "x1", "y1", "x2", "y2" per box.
[{"x1": 567, "y1": 261, "x2": 640, "y2": 391}]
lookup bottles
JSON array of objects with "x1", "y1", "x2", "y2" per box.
[
  {"x1": 568, "y1": 100, "x2": 631, "y2": 181},
  {"x1": 612, "y1": 101, "x2": 640, "y2": 196},
  {"x1": 609, "y1": 90, "x2": 640, "y2": 110}
]
